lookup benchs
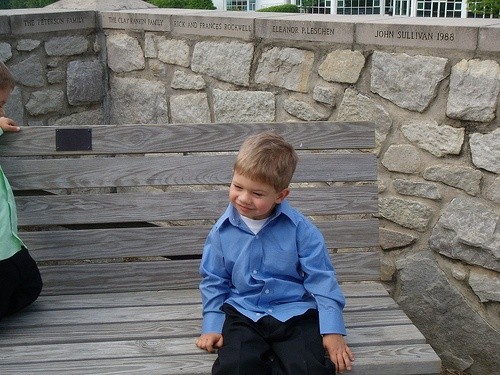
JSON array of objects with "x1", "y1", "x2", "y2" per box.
[{"x1": 0, "y1": 120, "x2": 448, "y2": 375}]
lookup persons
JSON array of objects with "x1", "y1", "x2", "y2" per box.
[
  {"x1": 0, "y1": 60, "x2": 44, "y2": 320},
  {"x1": 195, "y1": 130, "x2": 354, "y2": 375}
]
